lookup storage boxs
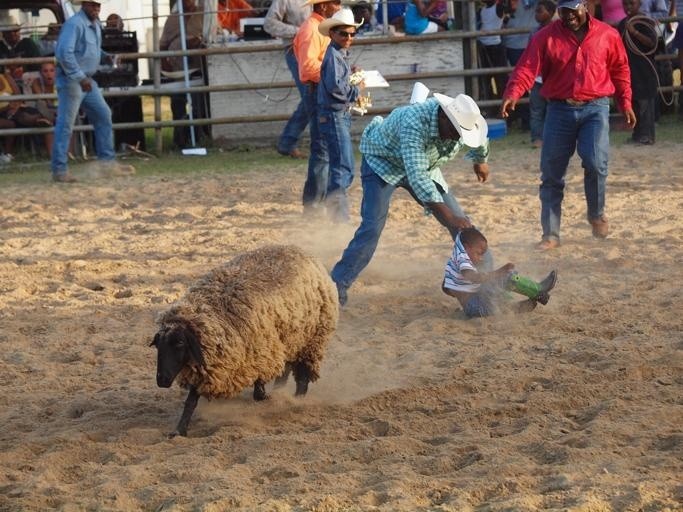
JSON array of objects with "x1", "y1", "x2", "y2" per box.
[{"x1": 485, "y1": 118, "x2": 507, "y2": 138}]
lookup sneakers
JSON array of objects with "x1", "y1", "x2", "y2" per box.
[
  {"x1": 531, "y1": 138, "x2": 544, "y2": 149},
  {"x1": 278, "y1": 141, "x2": 307, "y2": 160},
  {"x1": 98, "y1": 157, "x2": 137, "y2": 178},
  {"x1": 51, "y1": 168, "x2": 78, "y2": 184}
]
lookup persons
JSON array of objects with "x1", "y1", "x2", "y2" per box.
[
  {"x1": 328, "y1": 93, "x2": 493, "y2": 309},
  {"x1": 441, "y1": 229, "x2": 557, "y2": 316}
]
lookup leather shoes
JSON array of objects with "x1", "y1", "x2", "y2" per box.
[
  {"x1": 533, "y1": 239, "x2": 559, "y2": 255},
  {"x1": 589, "y1": 213, "x2": 610, "y2": 238}
]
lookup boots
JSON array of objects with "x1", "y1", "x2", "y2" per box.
[
  {"x1": 514, "y1": 266, "x2": 556, "y2": 307},
  {"x1": 503, "y1": 297, "x2": 538, "y2": 315}
]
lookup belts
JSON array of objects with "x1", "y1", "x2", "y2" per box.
[{"x1": 547, "y1": 95, "x2": 609, "y2": 102}]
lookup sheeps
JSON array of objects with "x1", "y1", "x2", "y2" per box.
[{"x1": 148, "y1": 244, "x2": 340, "y2": 440}]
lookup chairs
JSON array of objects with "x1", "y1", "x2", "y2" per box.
[{"x1": 21, "y1": 70, "x2": 89, "y2": 162}]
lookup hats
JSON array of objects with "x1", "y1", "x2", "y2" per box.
[
  {"x1": 300, "y1": 1, "x2": 334, "y2": 7},
  {"x1": 317, "y1": 8, "x2": 366, "y2": 37},
  {"x1": 433, "y1": 92, "x2": 490, "y2": 146},
  {"x1": 556, "y1": 0, "x2": 587, "y2": 12}
]
